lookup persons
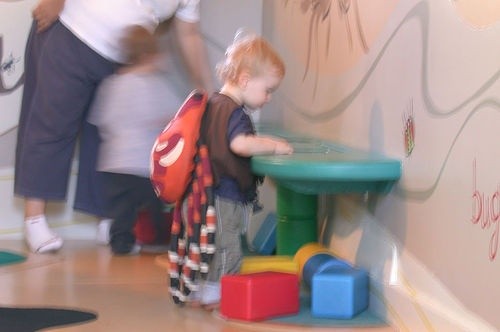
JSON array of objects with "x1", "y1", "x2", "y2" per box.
[
  {"x1": 86, "y1": 25, "x2": 197, "y2": 254},
  {"x1": 168, "y1": 25, "x2": 293, "y2": 305},
  {"x1": 14, "y1": 0, "x2": 213, "y2": 254}
]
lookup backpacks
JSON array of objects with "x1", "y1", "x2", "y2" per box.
[{"x1": 151, "y1": 87, "x2": 212, "y2": 209}]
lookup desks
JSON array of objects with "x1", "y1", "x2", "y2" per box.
[{"x1": 251, "y1": 131, "x2": 402, "y2": 256}]
